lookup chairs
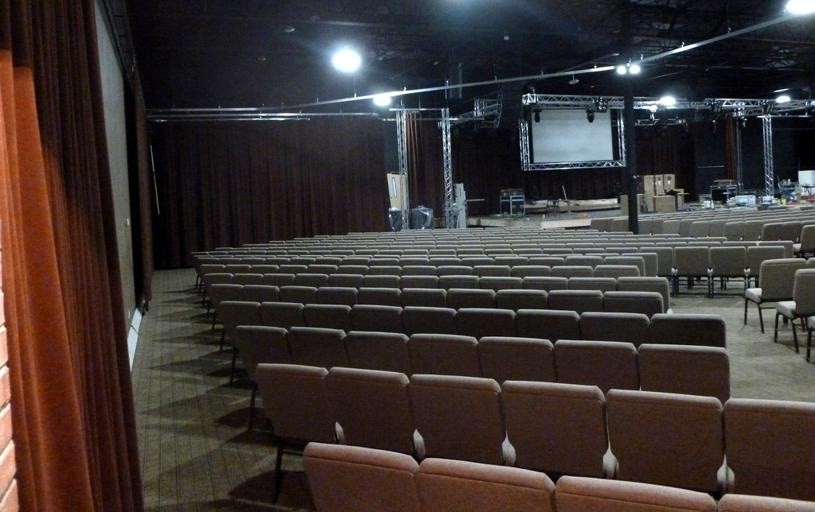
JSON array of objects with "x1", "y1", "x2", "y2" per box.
[{"x1": 189, "y1": 201, "x2": 815, "y2": 511}]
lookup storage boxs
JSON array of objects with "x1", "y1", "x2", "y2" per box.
[{"x1": 619, "y1": 173, "x2": 685, "y2": 216}]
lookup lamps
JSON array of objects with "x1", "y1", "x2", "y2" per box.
[{"x1": 526, "y1": 108, "x2": 595, "y2": 125}]
